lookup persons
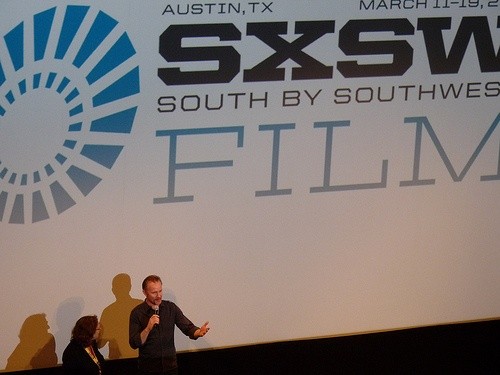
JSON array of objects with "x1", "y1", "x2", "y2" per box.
[
  {"x1": 61, "y1": 314, "x2": 110, "y2": 375},
  {"x1": 128, "y1": 274, "x2": 211, "y2": 374}
]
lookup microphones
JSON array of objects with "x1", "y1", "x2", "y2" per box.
[{"x1": 153, "y1": 305, "x2": 159, "y2": 327}]
